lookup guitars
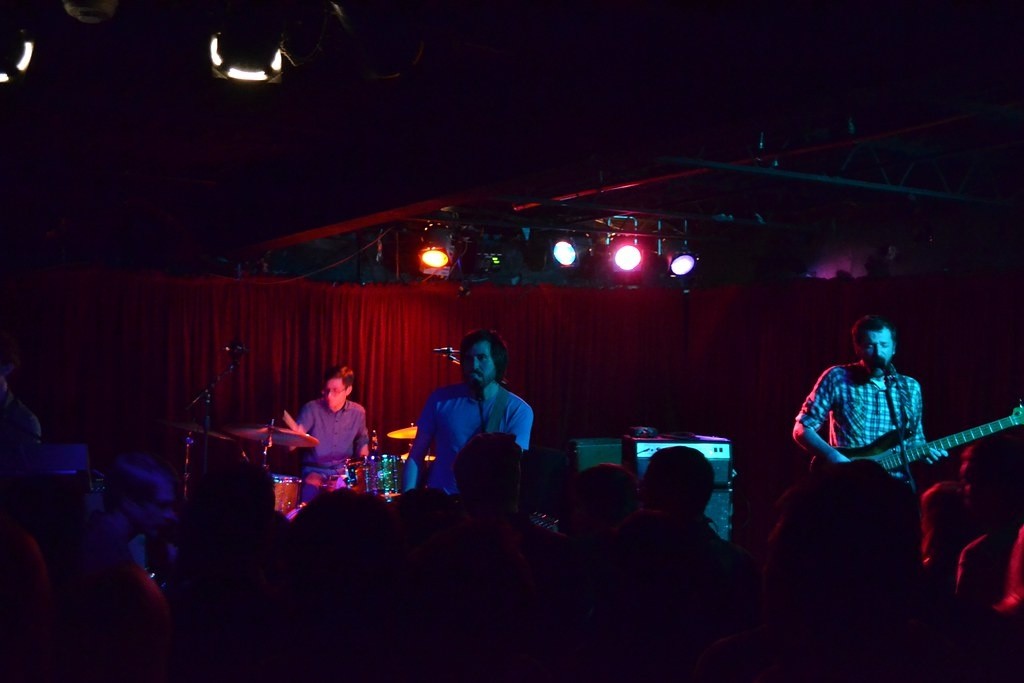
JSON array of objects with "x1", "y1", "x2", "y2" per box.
[{"x1": 803, "y1": 402, "x2": 1023, "y2": 485}]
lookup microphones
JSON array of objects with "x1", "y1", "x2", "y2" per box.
[
  {"x1": 432, "y1": 347, "x2": 460, "y2": 355},
  {"x1": 223, "y1": 345, "x2": 248, "y2": 353},
  {"x1": 873, "y1": 358, "x2": 896, "y2": 381}
]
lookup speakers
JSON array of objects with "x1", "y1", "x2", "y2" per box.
[
  {"x1": 704, "y1": 488, "x2": 733, "y2": 543},
  {"x1": 563, "y1": 438, "x2": 623, "y2": 475}
]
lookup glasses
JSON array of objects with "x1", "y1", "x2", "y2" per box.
[{"x1": 321, "y1": 386, "x2": 346, "y2": 397}]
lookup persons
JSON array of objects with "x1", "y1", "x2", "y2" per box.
[
  {"x1": 403, "y1": 328, "x2": 533, "y2": 491},
  {"x1": 295, "y1": 366, "x2": 368, "y2": 502},
  {"x1": 791, "y1": 315, "x2": 949, "y2": 480},
  {"x1": 0, "y1": 332, "x2": 41, "y2": 478},
  {"x1": 0, "y1": 432, "x2": 1024, "y2": 683}
]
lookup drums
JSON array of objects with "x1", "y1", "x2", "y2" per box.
[
  {"x1": 346, "y1": 456, "x2": 402, "y2": 496},
  {"x1": 259, "y1": 472, "x2": 303, "y2": 515}
]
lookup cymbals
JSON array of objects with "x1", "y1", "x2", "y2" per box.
[
  {"x1": 223, "y1": 426, "x2": 320, "y2": 448},
  {"x1": 162, "y1": 419, "x2": 231, "y2": 441},
  {"x1": 386, "y1": 425, "x2": 419, "y2": 440}
]
